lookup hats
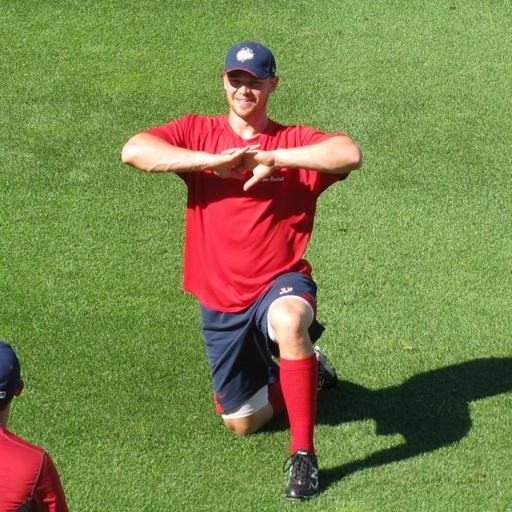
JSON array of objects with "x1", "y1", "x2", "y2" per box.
[
  {"x1": 221, "y1": 42, "x2": 276, "y2": 79},
  {"x1": 0, "y1": 340, "x2": 20, "y2": 404}
]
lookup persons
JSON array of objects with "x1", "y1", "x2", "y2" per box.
[
  {"x1": 0, "y1": 342, "x2": 69, "y2": 512},
  {"x1": 121, "y1": 42, "x2": 362, "y2": 499}
]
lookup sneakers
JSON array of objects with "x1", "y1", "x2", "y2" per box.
[
  {"x1": 313, "y1": 346, "x2": 337, "y2": 391},
  {"x1": 284, "y1": 449, "x2": 319, "y2": 499}
]
